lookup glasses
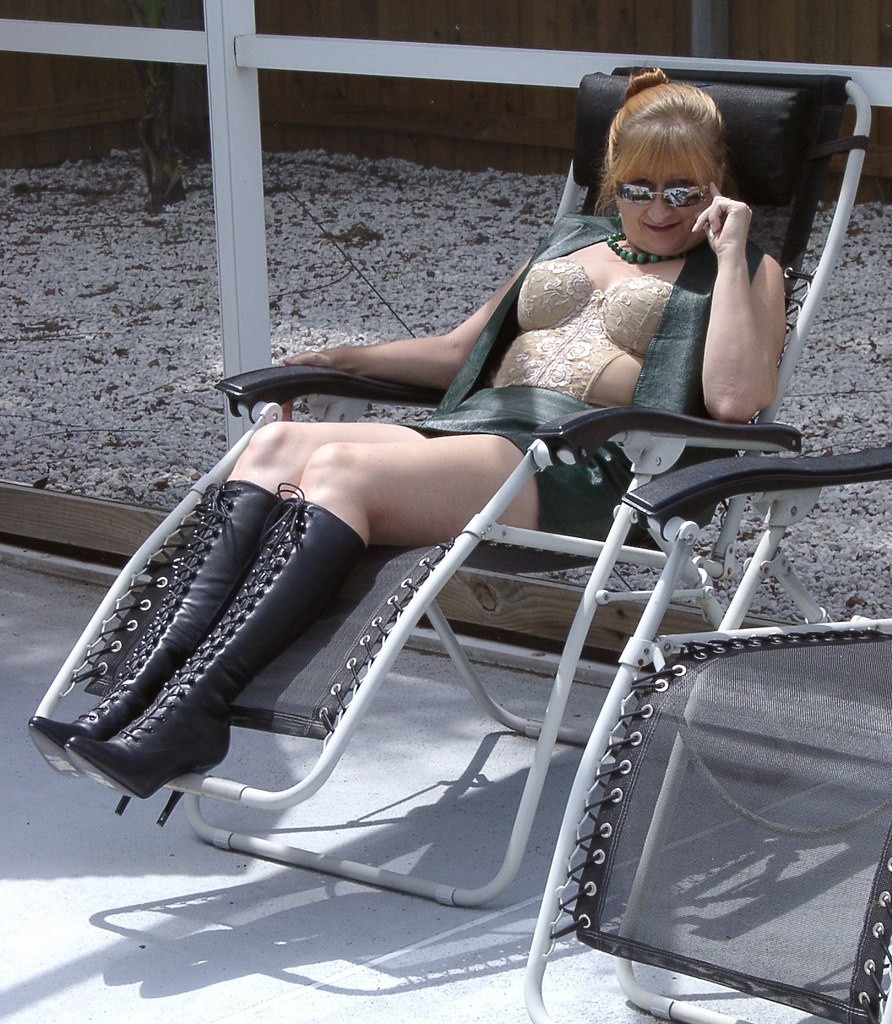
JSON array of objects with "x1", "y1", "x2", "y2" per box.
[{"x1": 613, "y1": 178, "x2": 710, "y2": 207}]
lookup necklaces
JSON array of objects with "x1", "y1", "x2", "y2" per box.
[{"x1": 605, "y1": 232, "x2": 688, "y2": 265}]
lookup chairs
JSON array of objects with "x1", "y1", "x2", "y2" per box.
[
  {"x1": 23, "y1": 55, "x2": 874, "y2": 911},
  {"x1": 520, "y1": 440, "x2": 892, "y2": 1024}
]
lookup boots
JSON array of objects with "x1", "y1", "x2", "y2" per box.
[
  {"x1": 67, "y1": 497, "x2": 365, "y2": 827},
  {"x1": 25, "y1": 480, "x2": 284, "y2": 816}
]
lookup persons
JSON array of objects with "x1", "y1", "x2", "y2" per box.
[{"x1": 22, "y1": 60, "x2": 789, "y2": 831}]
